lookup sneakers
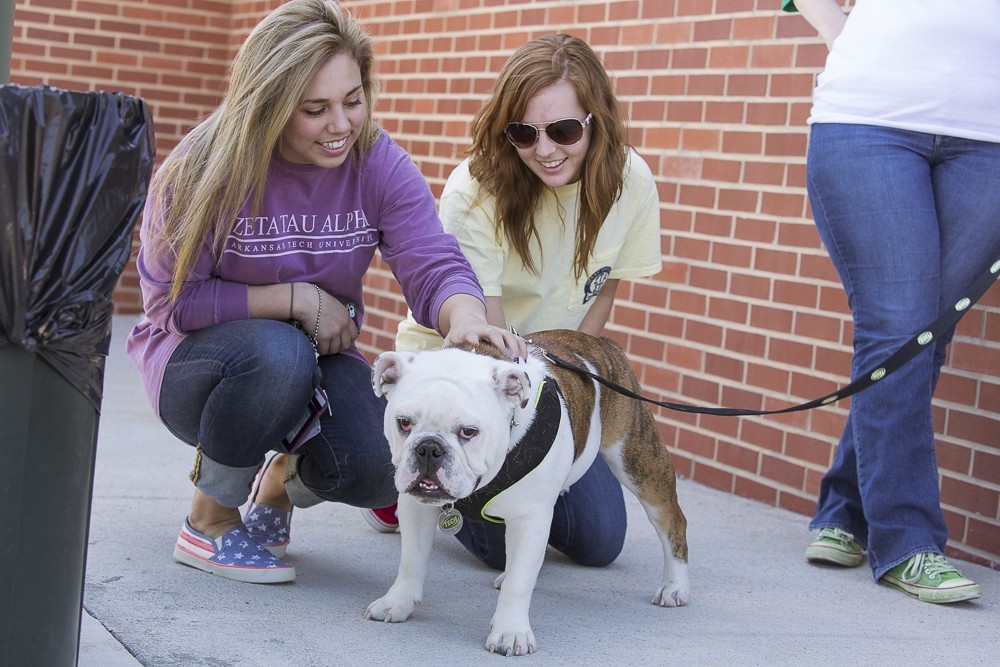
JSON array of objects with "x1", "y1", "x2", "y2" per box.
[
  {"x1": 806, "y1": 521, "x2": 865, "y2": 566},
  {"x1": 879, "y1": 548, "x2": 981, "y2": 605}
]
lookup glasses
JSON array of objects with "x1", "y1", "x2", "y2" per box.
[{"x1": 502, "y1": 112, "x2": 593, "y2": 149}]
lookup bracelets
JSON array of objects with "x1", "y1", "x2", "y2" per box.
[
  {"x1": 309, "y1": 284, "x2": 323, "y2": 347},
  {"x1": 290, "y1": 282, "x2": 294, "y2": 319}
]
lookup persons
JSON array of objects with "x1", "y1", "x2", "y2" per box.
[
  {"x1": 362, "y1": 34, "x2": 662, "y2": 571},
  {"x1": 126, "y1": 0, "x2": 528, "y2": 581},
  {"x1": 782, "y1": 0, "x2": 1000, "y2": 602}
]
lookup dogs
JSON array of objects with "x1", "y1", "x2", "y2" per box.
[{"x1": 372, "y1": 329, "x2": 690, "y2": 657}]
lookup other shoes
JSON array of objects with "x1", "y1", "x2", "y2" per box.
[
  {"x1": 241, "y1": 451, "x2": 293, "y2": 558},
  {"x1": 172, "y1": 510, "x2": 296, "y2": 582},
  {"x1": 362, "y1": 504, "x2": 399, "y2": 533}
]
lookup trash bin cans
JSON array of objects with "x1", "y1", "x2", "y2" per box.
[{"x1": 1, "y1": 83, "x2": 155, "y2": 667}]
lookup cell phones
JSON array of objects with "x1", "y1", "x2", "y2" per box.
[{"x1": 293, "y1": 303, "x2": 360, "y2": 336}]
